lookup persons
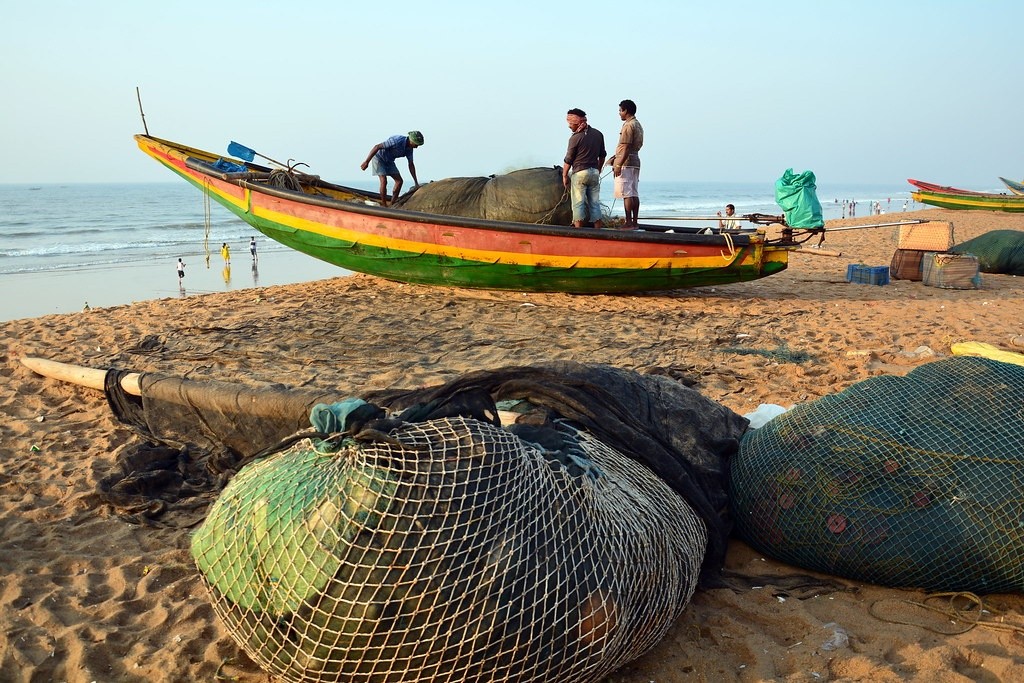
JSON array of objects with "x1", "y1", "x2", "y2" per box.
[
  {"x1": 221, "y1": 243, "x2": 230, "y2": 264},
  {"x1": 563, "y1": 108, "x2": 606, "y2": 229},
  {"x1": 717, "y1": 204, "x2": 742, "y2": 230},
  {"x1": 177, "y1": 258, "x2": 186, "y2": 279},
  {"x1": 250, "y1": 237, "x2": 257, "y2": 260},
  {"x1": 613, "y1": 99, "x2": 642, "y2": 230},
  {"x1": 361, "y1": 131, "x2": 424, "y2": 207}
]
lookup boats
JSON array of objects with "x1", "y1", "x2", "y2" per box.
[
  {"x1": 907, "y1": 178, "x2": 973, "y2": 195},
  {"x1": 910, "y1": 190, "x2": 1024, "y2": 213},
  {"x1": 1001, "y1": 176, "x2": 1024, "y2": 196},
  {"x1": 132, "y1": 132, "x2": 789, "y2": 294}
]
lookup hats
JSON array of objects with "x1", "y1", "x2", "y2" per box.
[{"x1": 408, "y1": 131, "x2": 425, "y2": 146}]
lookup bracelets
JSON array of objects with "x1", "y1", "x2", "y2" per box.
[{"x1": 616, "y1": 165, "x2": 622, "y2": 168}]
